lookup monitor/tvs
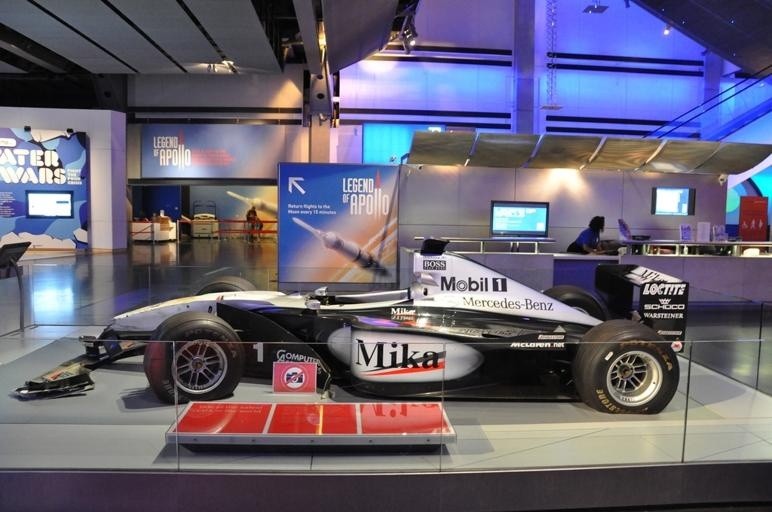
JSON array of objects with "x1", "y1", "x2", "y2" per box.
[
  {"x1": 0, "y1": 242, "x2": 31, "y2": 266},
  {"x1": 651, "y1": 187, "x2": 696, "y2": 216},
  {"x1": 490, "y1": 199, "x2": 550, "y2": 238},
  {"x1": 25, "y1": 191, "x2": 73, "y2": 218}
]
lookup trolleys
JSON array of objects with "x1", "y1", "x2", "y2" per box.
[{"x1": 190, "y1": 198, "x2": 221, "y2": 240}]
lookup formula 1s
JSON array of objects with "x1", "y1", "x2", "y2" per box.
[{"x1": 8, "y1": 237, "x2": 691, "y2": 416}]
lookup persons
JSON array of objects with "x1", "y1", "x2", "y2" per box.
[
  {"x1": 245, "y1": 206, "x2": 258, "y2": 245},
  {"x1": 255, "y1": 217, "x2": 264, "y2": 244},
  {"x1": 565, "y1": 215, "x2": 607, "y2": 254}
]
